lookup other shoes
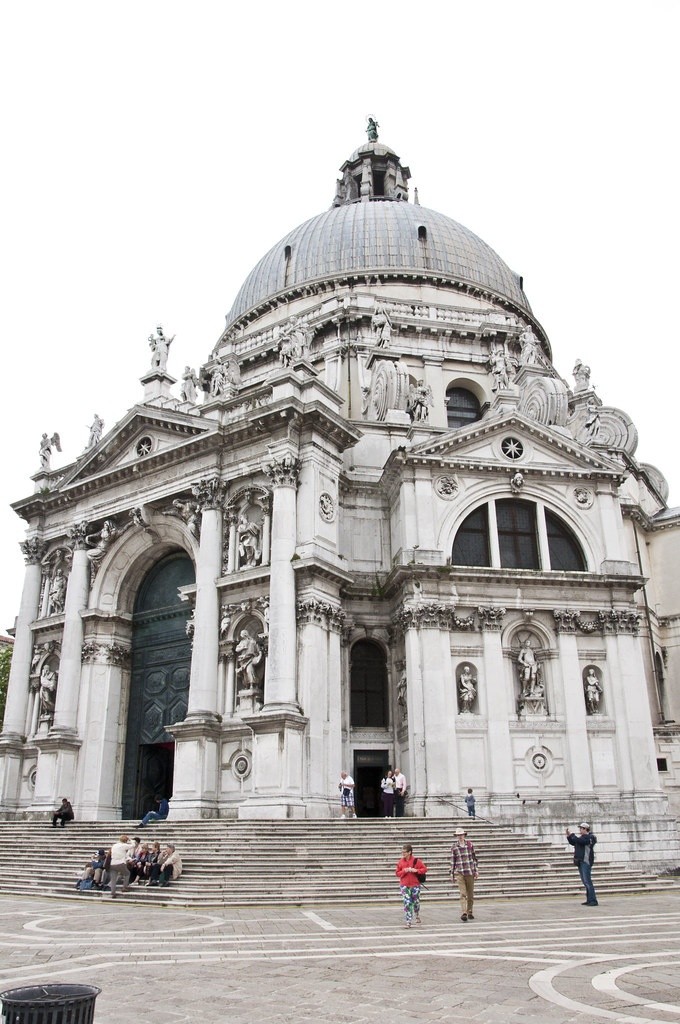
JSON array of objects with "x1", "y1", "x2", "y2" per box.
[
  {"x1": 133, "y1": 825, "x2": 143, "y2": 828},
  {"x1": 404, "y1": 918, "x2": 421, "y2": 929},
  {"x1": 111, "y1": 894, "x2": 116, "y2": 899},
  {"x1": 340, "y1": 814, "x2": 345, "y2": 818},
  {"x1": 49, "y1": 825, "x2": 65, "y2": 828},
  {"x1": 74, "y1": 878, "x2": 111, "y2": 891},
  {"x1": 353, "y1": 813, "x2": 357, "y2": 819},
  {"x1": 129, "y1": 882, "x2": 169, "y2": 887},
  {"x1": 385, "y1": 816, "x2": 392, "y2": 819},
  {"x1": 582, "y1": 901, "x2": 598, "y2": 906},
  {"x1": 121, "y1": 888, "x2": 129, "y2": 892},
  {"x1": 461, "y1": 913, "x2": 474, "y2": 921}
]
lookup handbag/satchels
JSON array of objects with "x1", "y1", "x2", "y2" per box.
[
  {"x1": 343, "y1": 788, "x2": 350, "y2": 796},
  {"x1": 396, "y1": 787, "x2": 408, "y2": 798},
  {"x1": 102, "y1": 849, "x2": 112, "y2": 872},
  {"x1": 413, "y1": 858, "x2": 426, "y2": 883},
  {"x1": 79, "y1": 880, "x2": 93, "y2": 890}
]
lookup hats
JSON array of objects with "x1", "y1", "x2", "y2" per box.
[
  {"x1": 578, "y1": 822, "x2": 590, "y2": 831},
  {"x1": 453, "y1": 828, "x2": 468, "y2": 838},
  {"x1": 95, "y1": 849, "x2": 105, "y2": 856}
]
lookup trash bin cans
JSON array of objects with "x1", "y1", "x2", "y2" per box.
[{"x1": 0, "y1": 983, "x2": 102, "y2": 1024}]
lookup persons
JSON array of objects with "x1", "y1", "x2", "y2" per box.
[
  {"x1": 395, "y1": 844, "x2": 428, "y2": 929},
  {"x1": 134, "y1": 794, "x2": 169, "y2": 827},
  {"x1": 519, "y1": 325, "x2": 541, "y2": 365},
  {"x1": 75, "y1": 835, "x2": 182, "y2": 898},
  {"x1": 450, "y1": 828, "x2": 479, "y2": 921},
  {"x1": 380, "y1": 768, "x2": 407, "y2": 818},
  {"x1": 460, "y1": 666, "x2": 477, "y2": 701},
  {"x1": 338, "y1": 770, "x2": 358, "y2": 819},
  {"x1": 396, "y1": 659, "x2": 407, "y2": 706},
  {"x1": 372, "y1": 301, "x2": 397, "y2": 348},
  {"x1": 366, "y1": 118, "x2": 382, "y2": 141},
  {"x1": 86, "y1": 414, "x2": 105, "y2": 447},
  {"x1": 85, "y1": 498, "x2": 201, "y2": 614},
  {"x1": 585, "y1": 669, "x2": 602, "y2": 713},
  {"x1": 235, "y1": 630, "x2": 266, "y2": 689},
  {"x1": 566, "y1": 822, "x2": 598, "y2": 906},
  {"x1": 181, "y1": 357, "x2": 230, "y2": 403},
  {"x1": 277, "y1": 315, "x2": 311, "y2": 368},
  {"x1": 489, "y1": 347, "x2": 509, "y2": 390},
  {"x1": 572, "y1": 358, "x2": 590, "y2": 385},
  {"x1": 238, "y1": 513, "x2": 262, "y2": 568},
  {"x1": 40, "y1": 665, "x2": 57, "y2": 715},
  {"x1": 39, "y1": 432, "x2": 51, "y2": 468},
  {"x1": 49, "y1": 799, "x2": 74, "y2": 828},
  {"x1": 465, "y1": 789, "x2": 475, "y2": 820},
  {"x1": 518, "y1": 640, "x2": 538, "y2": 696},
  {"x1": 49, "y1": 568, "x2": 68, "y2": 614},
  {"x1": 148, "y1": 327, "x2": 176, "y2": 372},
  {"x1": 413, "y1": 379, "x2": 427, "y2": 421}
]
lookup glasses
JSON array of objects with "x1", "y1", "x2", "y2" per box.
[{"x1": 401, "y1": 850, "x2": 409, "y2": 853}]
